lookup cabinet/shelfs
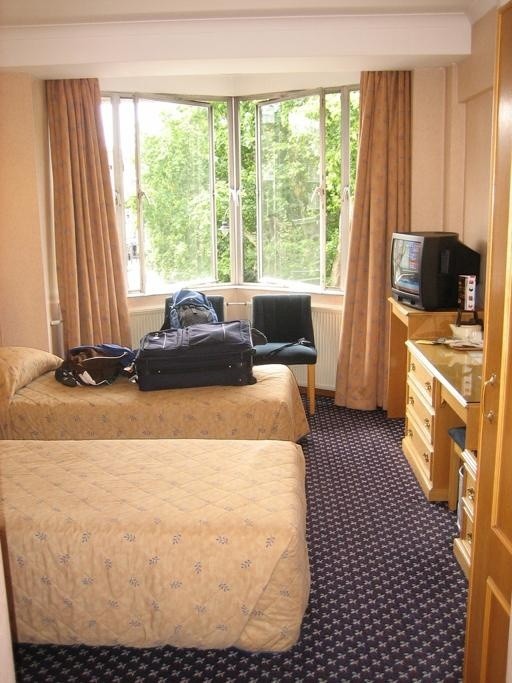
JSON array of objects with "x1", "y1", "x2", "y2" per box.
[{"x1": 388, "y1": 297, "x2": 486, "y2": 587}]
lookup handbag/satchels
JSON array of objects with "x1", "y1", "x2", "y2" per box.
[{"x1": 56, "y1": 342, "x2": 129, "y2": 386}]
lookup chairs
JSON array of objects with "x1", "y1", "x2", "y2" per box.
[{"x1": 165, "y1": 296, "x2": 318, "y2": 413}]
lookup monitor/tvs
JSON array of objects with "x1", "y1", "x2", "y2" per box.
[{"x1": 390, "y1": 231, "x2": 481, "y2": 311}]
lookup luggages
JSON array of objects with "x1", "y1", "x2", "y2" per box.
[{"x1": 132, "y1": 318, "x2": 257, "y2": 393}]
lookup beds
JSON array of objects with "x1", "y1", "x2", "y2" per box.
[
  {"x1": 0, "y1": 438, "x2": 316, "y2": 663},
  {"x1": 1, "y1": 365, "x2": 307, "y2": 448}
]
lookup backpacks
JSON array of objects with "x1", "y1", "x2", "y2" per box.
[{"x1": 168, "y1": 287, "x2": 219, "y2": 329}]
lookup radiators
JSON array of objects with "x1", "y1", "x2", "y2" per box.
[{"x1": 295, "y1": 304, "x2": 346, "y2": 392}]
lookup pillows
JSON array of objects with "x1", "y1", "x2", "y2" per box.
[{"x1": 1, "y1": 346, "x2": 62, "y2": 403}]
